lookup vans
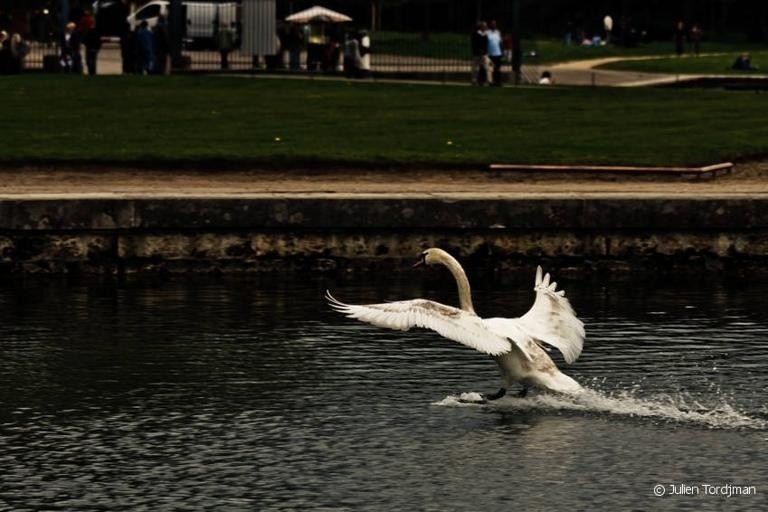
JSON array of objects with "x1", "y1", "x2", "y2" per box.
[{"x1": 126, "y1": 0, "x2": 238, "y2": 50}]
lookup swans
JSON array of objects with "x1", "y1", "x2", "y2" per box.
[{"x1": 324, "y1": 245, "x2": 589, "y2": 402}]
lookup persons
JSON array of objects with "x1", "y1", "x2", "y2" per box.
[
  {"x1": 1, "y1": 1, "x2": 170, "y2": 76},
  {"x1": 478, "y1": 19, "x2": 506, "y2": 88},
  {"x1": 672, "y1": 21, "x2": 687, "y2": 56},
  {"x1": 732, "y1": 51, "x2": 760, "y2": 72},
  {"x1": 281, "y1": 23, "x2": 374, "y2": 73},
  {"x1": 537, "y1": 70, "x2": 555, "y2": 85},
  {"x1": 228, "y1": 21, "x2": 239, "y2": 43},
  {"x1": 219, "y1": 21, "x2": 232, "y2": 52},
  {"x1": 470, "y1": 19, "x2": 496, "y2": 87},
  {"x1": 690, "y1": 24, "x2": 704, "y2": 53}
]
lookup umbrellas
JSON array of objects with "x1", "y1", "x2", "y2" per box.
[{"x1": 284, "y1": 5, "x2": 355, "y2": 26}]
